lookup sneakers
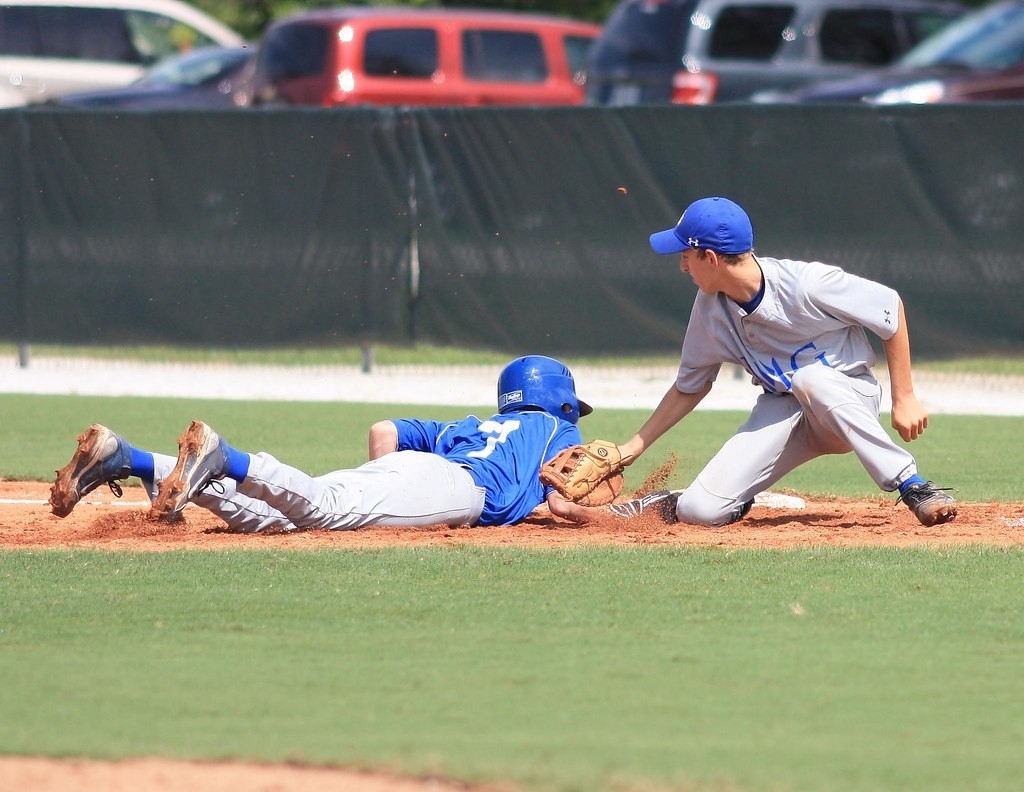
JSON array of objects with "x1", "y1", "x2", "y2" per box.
[
  {"x1": 895, "y1": 480, "x2": 959, "y2": 527},
  {"x1": 47, "y1": 422, "x2": 131, "y2": 518},
  {"x1": 146, "y1": 420, "x2": 229, "y2": 520},
  {"x1": 740, "y1": 497, "x2": 755, "y2": 518}
]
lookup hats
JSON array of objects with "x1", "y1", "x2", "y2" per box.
[{"x1": 649, "y1": 196, "x2": 753, "y2": 255}]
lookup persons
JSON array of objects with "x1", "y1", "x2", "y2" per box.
[
  {"x1": 538, "y1": 196, "x2": 957, "y2": 530},
  {"x1": 47, "y1": 355, "x2": 684, "y2": 535}
]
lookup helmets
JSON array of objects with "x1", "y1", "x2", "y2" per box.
[{"x1": 497, "y1": 355, "x2": 593, "y2": 424}]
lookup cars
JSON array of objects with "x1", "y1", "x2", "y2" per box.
[
  {"x1": 17, "y1": 44, "x2": 290, "y2": 106},
  {"x1": 253, "y1": 6, "x2": 719, "y2": 104},
  {"x1": 753, "y1": 0, "x2": 1024, "y2": 101},
  {"x1": 585, "y1": 0, "x2": 970, "y2": 107},
  {"x1": 0, "y1": 0, "x2": 252, "y2": 109}
]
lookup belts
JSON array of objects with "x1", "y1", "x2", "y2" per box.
[{"x1": 464, "y1": 466, "x2": 485, "y2": 487}]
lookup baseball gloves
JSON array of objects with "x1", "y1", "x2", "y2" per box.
[{"x1": 539, "y1": 440, "x2": 628, "y2": 509}]
